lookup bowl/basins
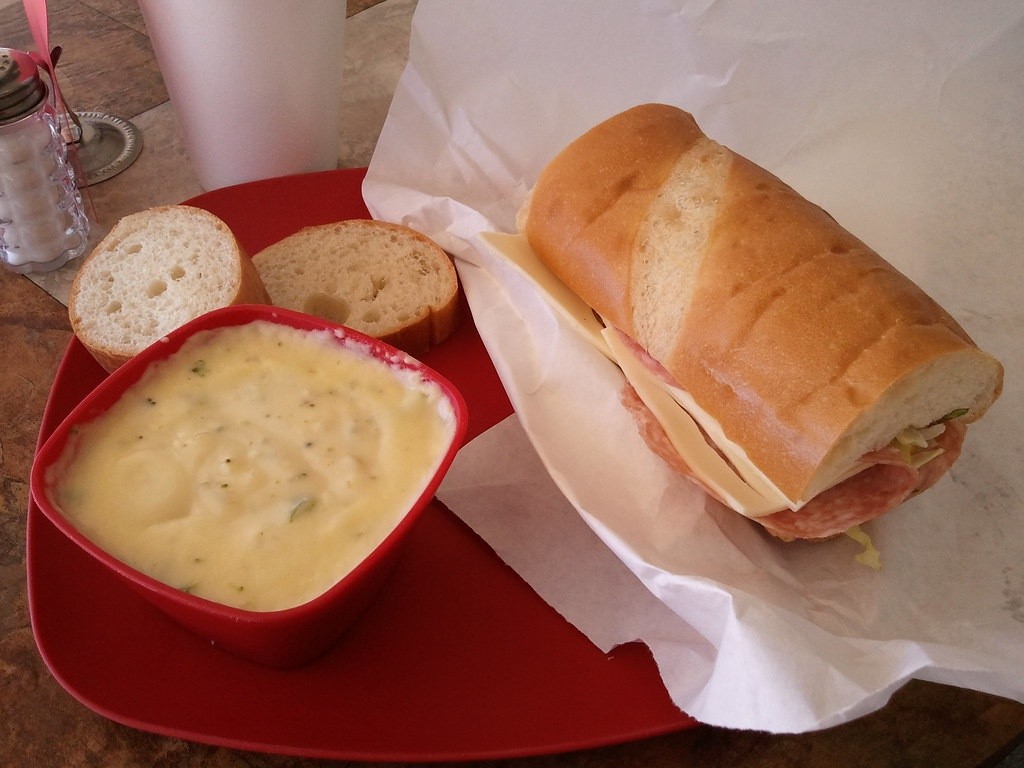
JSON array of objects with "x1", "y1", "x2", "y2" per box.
[{"x1": 30, "y1": 304, "x2": 468, "y2": 668}]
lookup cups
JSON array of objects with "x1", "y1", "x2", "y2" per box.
[{"x1": 133, "y1": 0, "x2": 349, "y2": 193}]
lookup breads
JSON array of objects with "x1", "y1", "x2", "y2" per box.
[
  {"x1": 256, "y1": 223, "x2": 459, "y2": 334},
  {"x1": 509, "y1": 105, "x2": 1005, "y2": 544},
  {"x1": 72, "y1": 209, "x2": 236, "y2": 361}
]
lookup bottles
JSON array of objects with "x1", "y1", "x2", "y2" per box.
[{"x1": 0, "y1": 48, "x2": 90, "y2": 273}]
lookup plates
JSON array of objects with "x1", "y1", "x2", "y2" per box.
[{"x1": 26, "y1": 167, "x2": 711, "y2": 762}]
